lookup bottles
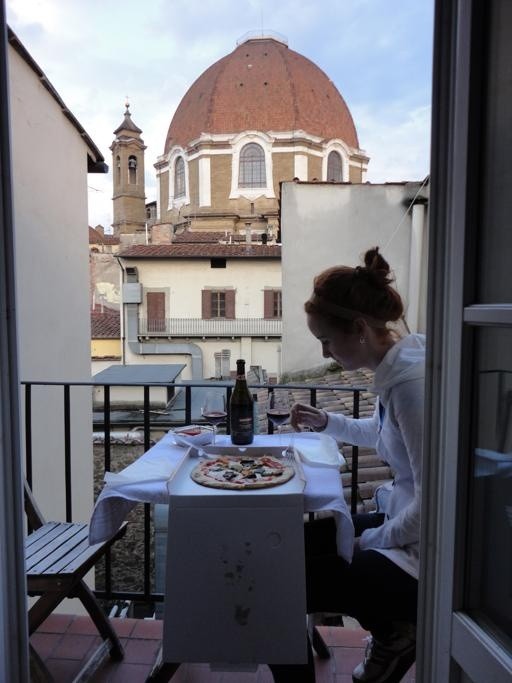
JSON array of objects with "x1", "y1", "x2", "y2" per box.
[{"x1": 229, "y1": 359, "x2": 255, "y2": 445}]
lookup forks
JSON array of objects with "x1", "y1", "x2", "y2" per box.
[{"x1": 281, "y1": 418, "x2": 300, "y2": 466}]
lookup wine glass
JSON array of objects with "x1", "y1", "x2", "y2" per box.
[
  {"x1": 201, "y1": 392, "x2": 228, "y2": 445},
  {"x1": 267, "y1": 391, "x2": 291, "y2": 445}
]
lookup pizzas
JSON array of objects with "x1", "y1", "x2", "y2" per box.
[{"x1": 190, "y1": 456, "x2": 294, "y2": 490}]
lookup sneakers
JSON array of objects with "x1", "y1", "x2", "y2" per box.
[{"x1": 352, "y1": 622, "x2": 417, "y2": 683}]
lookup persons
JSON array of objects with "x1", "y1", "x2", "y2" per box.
[{"x1": 289, "y1": 243, "x2": 429, "y2": 682}]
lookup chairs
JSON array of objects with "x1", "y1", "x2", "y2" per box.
[{"x1": 22, "y1": 474, "x2": 129, "y2": 681}]
